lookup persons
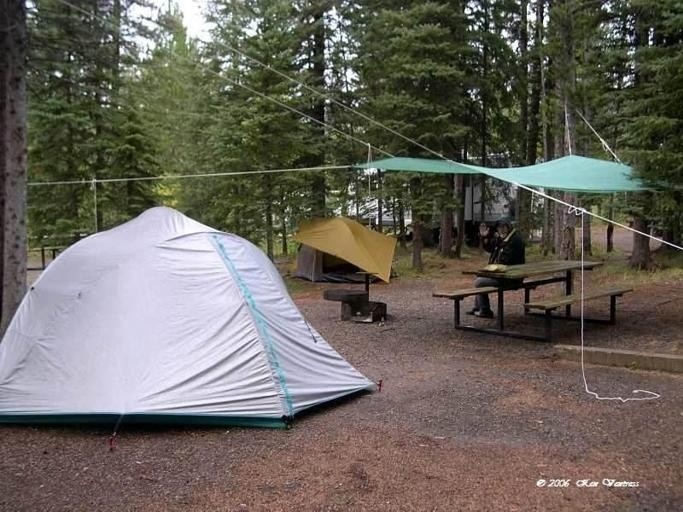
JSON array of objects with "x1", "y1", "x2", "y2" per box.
[{"x1": 466, "y1": 217, "x2": 525, "y2": 317}]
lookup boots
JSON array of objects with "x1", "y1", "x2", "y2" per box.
[
  {"x1": 465, "y1": 306, "x2": 480, "y2": 315},
  {"x1": 474, "y1": 306, "x2": 491, "y2": 317}
]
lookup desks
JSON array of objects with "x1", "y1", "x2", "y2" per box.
[{"x1": 460, "y1": 260, "x2": 604, "y2": 342}]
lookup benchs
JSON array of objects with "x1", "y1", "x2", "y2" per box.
[
  {"x1": 27, "y1": 246, "x2": 68, "y2": 270},
  {"x1": 433, "y1": 281, "x2": 536, "y2": 327},
  {"x1": 524, "y1": 286, "x2": 633, "y2": 342}
]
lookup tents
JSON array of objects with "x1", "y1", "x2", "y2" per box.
[
  {"x1": 291, "y1": 212, "x2": 397, "y2": 285},
  {"x1": 0, "y1": 205, "x2": 379, "y2": 427}
]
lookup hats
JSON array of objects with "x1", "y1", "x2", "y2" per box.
[{"x1": 495, "y1": 218, "x2": 513, "y2": 230}]
